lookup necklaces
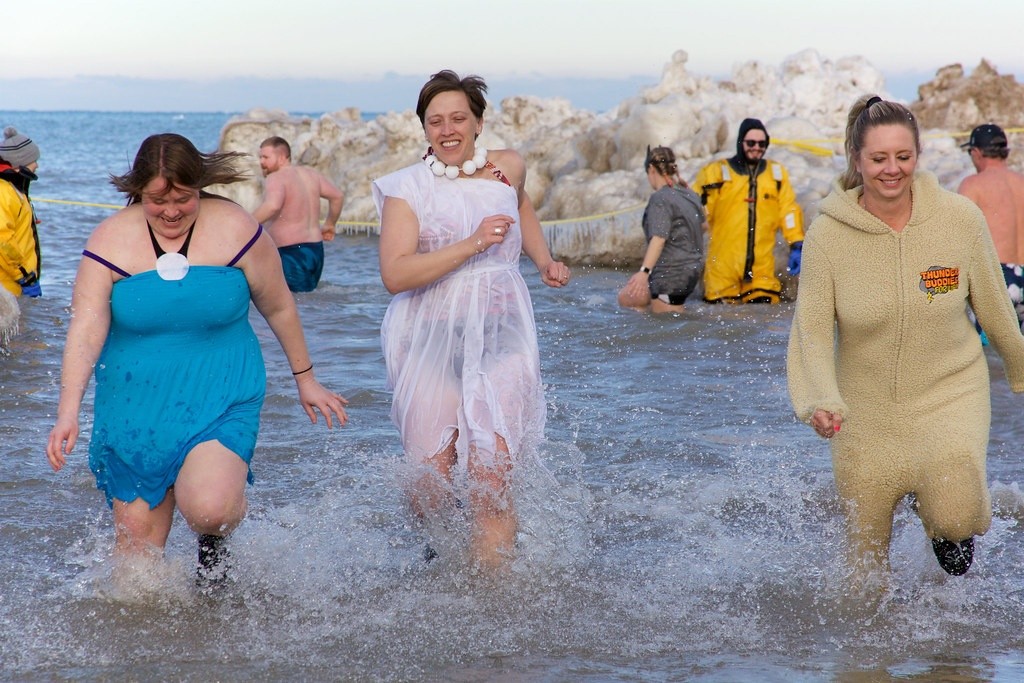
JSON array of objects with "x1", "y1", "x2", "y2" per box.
[
  {"x1": 145, "y1": 217, "x2": 196, "y2": 282},
  {"x1": 422, "y1": 145, "x2": 489, "y2": 180}
]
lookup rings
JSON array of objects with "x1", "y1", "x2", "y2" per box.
[
  {"x1": 564, "y1": 275, "x2": 568, "y2": 279},
  {"x1": 494, "y1": 226, "x2": 501, "y2": 236}
]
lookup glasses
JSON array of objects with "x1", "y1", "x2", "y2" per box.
[
  {"x1": 967, "y1": 147, "x2": 973, "y2": 155},
  {"x1": 743, "y1": 139, "x2": 767, "y2": 148}
]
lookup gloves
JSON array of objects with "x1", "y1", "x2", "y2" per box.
[
  {"x1": 787, "y1": 240, "x2": 804, "y2": 277},
  {"x1": 15, "y1": 271, "x2": 43, "y2": 298}
]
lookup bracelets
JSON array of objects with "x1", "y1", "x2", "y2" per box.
[{"x1": 291, "y1": 364, "x2": 313, "y2": 375}]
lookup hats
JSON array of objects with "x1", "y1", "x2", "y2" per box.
[
  {"x1": 0, "y1": 127, "x2": 40, "y2": 169},
  {"x1": 959, "y1": 124, "x2": 1008, "y2": 148}
]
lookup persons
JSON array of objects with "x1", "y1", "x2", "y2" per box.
[
  {"x1": 957, "y1": 124, "x2": 1024, "y2": 346},
  {"x1": 616, "y1": 144, "x2": 709, "y2": 319},
  {"x1": 369, "y1": 70, "x2": 573, "y2": 578},
  {"x1": 690, "y1": 118, "x2": 805, "y2": 305},
  {"x1": 786, "y1": 93, "x2": 1024, "y2": 601},
  {"x1": 254, "y1": 136, "x2": 344, "y2": 294},
  {"x1": 44, "y1": 132, "x2": 351, "y2": 598},
  {"x1": 0, "y1": 126, "x2": 45, "y2": 298}
]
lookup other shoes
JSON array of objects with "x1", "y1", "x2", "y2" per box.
[
  {"x1": 931, "y1": 535, "x2": 975, "y2": 575},
  {"x1": 195, "y1": 532, "x2": 230, "y2": 591}
]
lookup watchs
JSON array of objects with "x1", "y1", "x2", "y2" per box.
[{"x1": 639, "y1": 266, "x2": 652, "y2": 274}]
